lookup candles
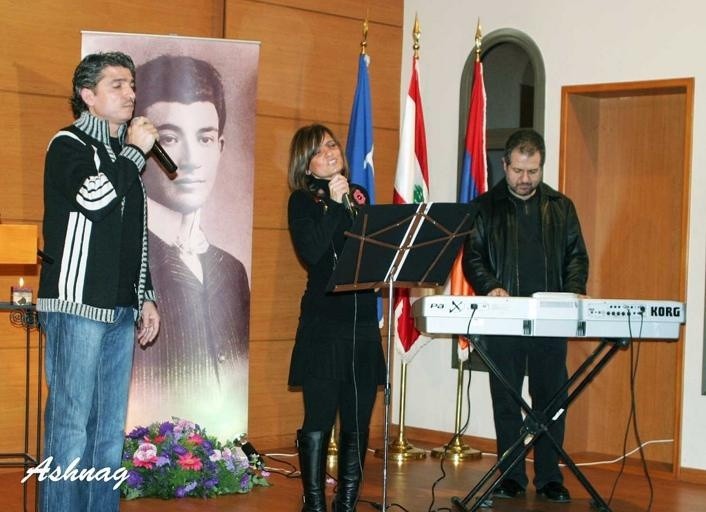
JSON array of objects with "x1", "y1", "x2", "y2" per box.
[{"x1": 11, "y1": 278, "x2": 34, "y2": 307}]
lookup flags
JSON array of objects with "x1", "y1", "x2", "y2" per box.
[
  {"x1": 347, "y1": 55, "x2": 384, "y2": 331},
  {"x1": 452, "y1": 57, "x2": 487, "y2": 361},
  {"x1": 390, "y1": 58, "x2": 433, "y2": 365}
]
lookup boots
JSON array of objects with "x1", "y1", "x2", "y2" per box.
[
  {"x1": 333, "y1": 428, "x2": 369, "y2": 510},
  {"x1": 296, "y1": 426, "x2": 331, "y2": 511}
]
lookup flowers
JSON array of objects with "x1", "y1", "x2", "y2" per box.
[{"x1": 120, "y1": 416, "x2": 271, "y2": 501}]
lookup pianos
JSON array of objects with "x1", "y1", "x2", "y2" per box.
[{"x1": 412, "y1": 291, "x2": 684, "y2": 342}]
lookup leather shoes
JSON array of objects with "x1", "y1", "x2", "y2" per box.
[
  {"x1": 494, "y1": 481, "x2": 525, "y2": 498},
  {"x1": 536, "y1": 481, "x2": 570, "y2": 503}
]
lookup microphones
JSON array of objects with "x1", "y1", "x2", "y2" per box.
[
  {"x1": 341, "y1": 193, "x2": 355, "y2": 216},
  {"x1": 153, "y1": 142, "x2": 178, "y2": 173}
]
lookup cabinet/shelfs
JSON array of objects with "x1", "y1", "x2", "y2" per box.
[{"x1": 1, "y1": 302, "x2": 42, "y2": 512}]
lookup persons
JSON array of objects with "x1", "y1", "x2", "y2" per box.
[
  {"x1": 130, "y1": 55, "x2": 251, "y2": 395},
  {"x1": 287, "y1": 125, "x2": 384, "y2": 512},
  {"x1": 463, "y1": 129, "x2": 588, "y2": 503},
  {"x1": 36, "y1": 52, "x2": 158, "y2": 510}
]
